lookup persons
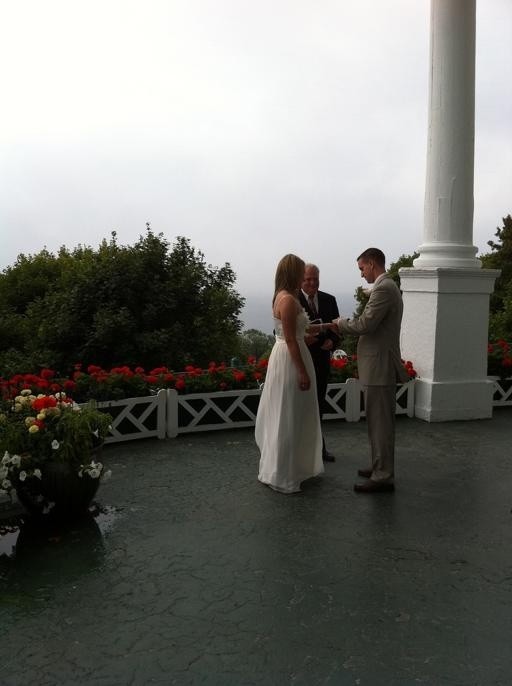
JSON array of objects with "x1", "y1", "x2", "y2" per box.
[
  {"x1": 254, "y1": 253, "x2": 341, "y2": 495},
  {"x1": 299, "y1": 262, "x2": 340, "y2": 464},
  {"x1": 332, "y1": 246, "x2": 408, "y2": 496}
]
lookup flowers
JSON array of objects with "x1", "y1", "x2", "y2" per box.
[{"x1": 0, "y1": 386, "x2": 112, "y2": 493}]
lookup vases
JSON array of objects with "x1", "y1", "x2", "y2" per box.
[{"x1": 14, "y1": 478, "x2": 99, "y2": 519}]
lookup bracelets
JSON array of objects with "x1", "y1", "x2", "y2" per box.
[{"x1": 321, "y1": 323, "x2": 326, "y2": 333}]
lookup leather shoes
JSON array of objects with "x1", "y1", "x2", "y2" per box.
[
  {"x1": 324, "y1": 451, "x2": 334, "y2": 461},
  {"x1": 355, "y1": 470, "x2": 395, "y2": 492}
]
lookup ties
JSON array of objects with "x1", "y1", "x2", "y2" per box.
[{"x1": 309, "y1": 295, "x2": 317, "y2": 316}]
